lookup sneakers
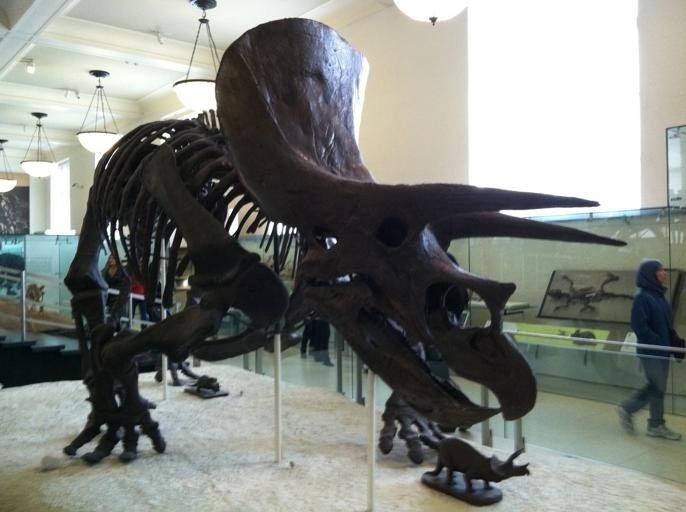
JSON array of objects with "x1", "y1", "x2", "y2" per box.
[
  {"x1": 645, "y1": 421, "x2": 681, "y2": 441},
  {"x1": 617, "y1": 403, "x2": 634, "y2": 434}
]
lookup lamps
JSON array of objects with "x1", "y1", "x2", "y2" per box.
[
  {"x1": 0, "y1": 70, "x2": 123, "y2": 192},
  {"x1": 173, "y1": 0, "x2": 227, "y2": 116}
]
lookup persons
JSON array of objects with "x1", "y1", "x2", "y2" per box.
[
  {"x1": 301, "y1": 312, "x2": 319, "y2": 359},
  {"x1": 99, "y1": 252, "x2": 129, "y2": 333},
  {"x1": 126, "y1": 256, "x2": 153, "y2": 331},
  {"x1": 612, "y1": 257, "x2": 685, "y2": 441},
  {"x1": 311, "y1": 318, "x2": 337, "y2": 368}
]
